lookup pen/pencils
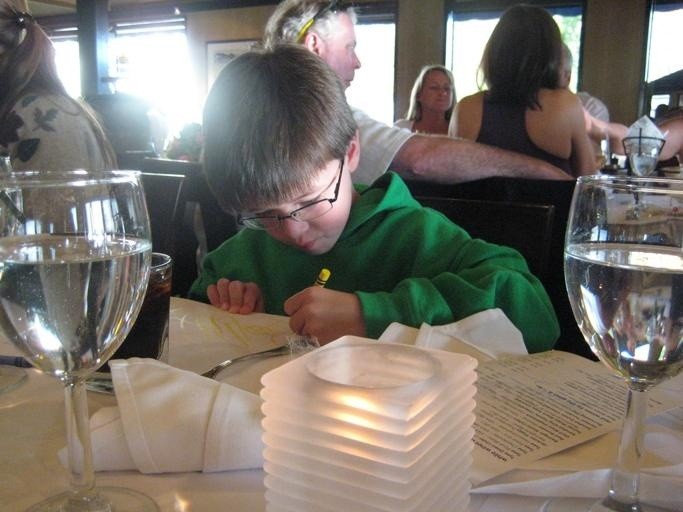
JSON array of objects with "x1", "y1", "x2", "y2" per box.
[{"x1": 313, "y1": 269, "x2": 330, "y2": 287}]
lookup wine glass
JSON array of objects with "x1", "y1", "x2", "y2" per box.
[
  {"x1": 0, "y1": 166, "x2": 164, "y2": 512},
  {"x1": 562, "y1": 171, "x2": 683, "y2": 512}
]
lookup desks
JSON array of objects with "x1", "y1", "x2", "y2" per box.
[{"x1": 0, "y1": 297, "x2": 682, "y2": 512}]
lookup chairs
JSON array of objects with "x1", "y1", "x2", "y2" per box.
[{"x1": 114, "y1": 145, "x2": 600, "y2": 359}]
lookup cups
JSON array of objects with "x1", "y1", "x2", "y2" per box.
[
  {"x1": 630, "y1": 143, "x2": 660, "y2": 178},
  {"x1": 95, "y1": 252, "x2": 173, "y2": 373}
]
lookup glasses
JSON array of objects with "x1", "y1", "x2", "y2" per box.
[{"x1": 235, "y1": 160, "x2": 346, "y2": 231}]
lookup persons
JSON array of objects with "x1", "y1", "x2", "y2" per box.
[
  {"x1": 165, "y1": 121, "x2": 204, "y2": 161},
  {"x1": 187, "y1": 34, "x2": 561, "y2": 356},
  {"x1": 446, "y1": 3, "x2": 605, "y2": 181},
  {"x1": 389, "y1": 64, "x2": 459, "y2": 137},
  {"x1": 193, "y1": 0, "x2": 579, "y2": 282},
  {"x1": 579, "y1": 100, "x2": 682, "y2": 167},
  {"x1": 1, "y1": 0, "x2": 129, "y2": 368},
  {"x1": 557, "y1": 38, "x2": 611, "y2": 168},
  {"x1": 564, "y1": 250, "x2": 683, "y2": 365}
]
